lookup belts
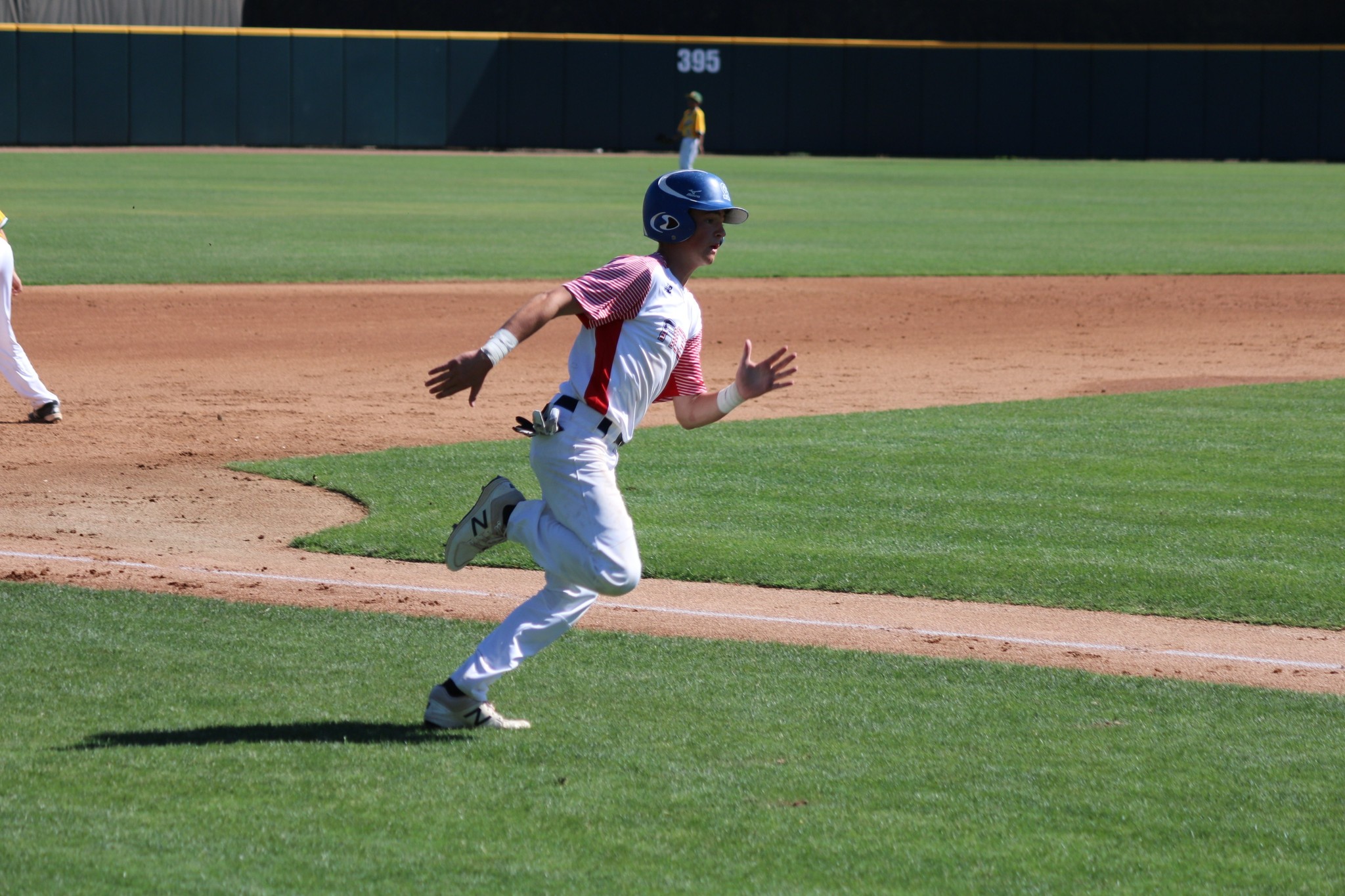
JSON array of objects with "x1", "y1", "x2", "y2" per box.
[{"x1": 555, "y1": 396, "x2": 626, "y2": 450}]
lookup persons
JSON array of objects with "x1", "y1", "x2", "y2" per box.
[
  {"x1": 0, "y1": 209, "x2": 63, "y2": 425},
  {"x1": 678, "y1": 92, "x2": 706, "y2": 169},
  {"x1": 423, "y1": 167, "x2": 800, "y2": 732}
]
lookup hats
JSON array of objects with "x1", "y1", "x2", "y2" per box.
[{"x1": 685, "y1": 89, "x2": 703, "y2": 104}]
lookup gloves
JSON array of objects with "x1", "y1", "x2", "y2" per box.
[{"x1": 511, "y1": 407, "x2": 561, "y2": 438}]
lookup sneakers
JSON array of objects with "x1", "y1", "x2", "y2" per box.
[
  {"x1": 443, "y1": 474, "x2": 526, "y2": 572},
  {"x1": 417, "y1": 684, "x2": 533, "y2": 734},
  {"x1": 27, "y1": 400, "x2": 63, "y2": 423}
]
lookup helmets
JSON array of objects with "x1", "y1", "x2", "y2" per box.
[{"x1": 641, "y1": 169, "x2": 750, "y2": 245}]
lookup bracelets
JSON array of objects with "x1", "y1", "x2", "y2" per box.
[
  {"x1": 717, "y1": 381, "x2": 747, "y2": 414},
  {"x1": 479, "y1": 328, "x2": 519, "y2": 368}
]
